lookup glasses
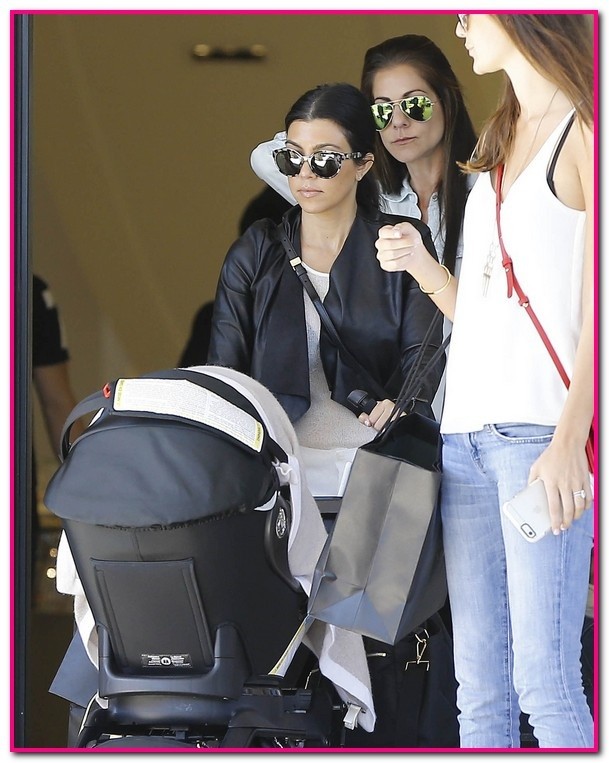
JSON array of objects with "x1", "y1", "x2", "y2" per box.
[
  {"x1": 455, "y1": 13, "x2": 471, "y2": 30},
  {"x1": 368, "y1": 94, "x2": 442, "y2": 132},
  {"x1": 273, "y1": 144, "x2": 363, "y2": 179}
]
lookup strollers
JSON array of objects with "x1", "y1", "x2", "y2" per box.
[{"x1": 43, "y1": 362, "x2": 383, "y2": 748}]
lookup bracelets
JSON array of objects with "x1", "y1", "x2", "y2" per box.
[{"x1": 419, "y1": 264, "x2": 452, "y2": 295}]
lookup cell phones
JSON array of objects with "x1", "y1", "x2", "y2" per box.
[{"x1": 504, "y1": 478, "x2": 552, "y2": 542}]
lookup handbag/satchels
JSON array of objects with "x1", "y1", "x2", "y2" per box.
[{"x1": 305, "y1": 310, "x2": 448, "y2": 644}]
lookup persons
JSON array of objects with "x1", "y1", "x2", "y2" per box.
[
  {"x1": 32, "y1": 272, "x2": 90, "y2": 457},
  {"x1": 179, "y1": 13, "x2": 595, "y2": 749}
]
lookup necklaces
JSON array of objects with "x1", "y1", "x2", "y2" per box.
[{"x1": 480, "y1": 86, "x2": 560, "y2": 297}]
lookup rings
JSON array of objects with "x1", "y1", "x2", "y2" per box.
[{"x1": 572, "y1": 489, "x2": 587, "y2": 499}]
